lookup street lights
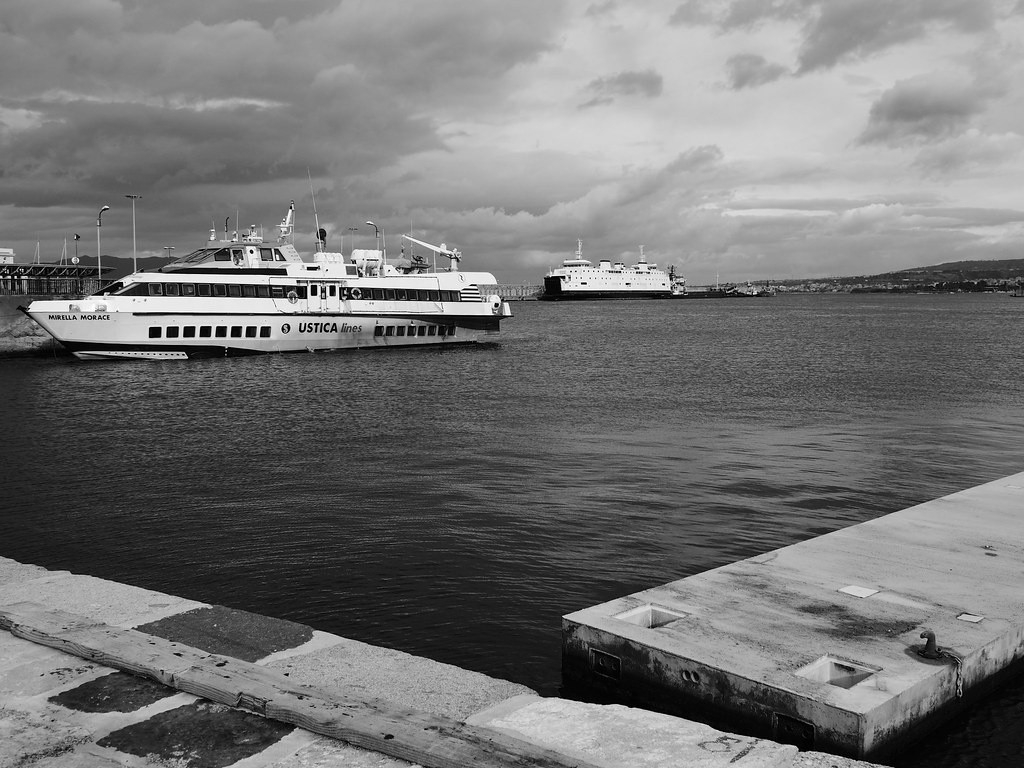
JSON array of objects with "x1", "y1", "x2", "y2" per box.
[
  {"x1": 124, "y1": 191, "x2": 142, "y2": 274},
  {"x1": 96, "y1": 202, "x2": 109, "y2": 289},
  {"x1": 73, "y1": 233, "x2": 79, "y2": 279},
  {"x1": 163, "y1": 245, "x2": 175, "y2": 266}
]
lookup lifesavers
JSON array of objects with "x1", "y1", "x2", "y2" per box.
[
  {"x1": 351, "y1": 287, "x2": 361, "y2": 299},
  {"x1": 287, "y1": 291, "x2": 298, "y2": 304}
]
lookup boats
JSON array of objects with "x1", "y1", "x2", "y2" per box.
[
  {"x1": 12, "y1": 159, "x2": 510, "y2": 359},
  {"x1": 542, "y1": 232, "x2": 673, "y2": 302}
]
